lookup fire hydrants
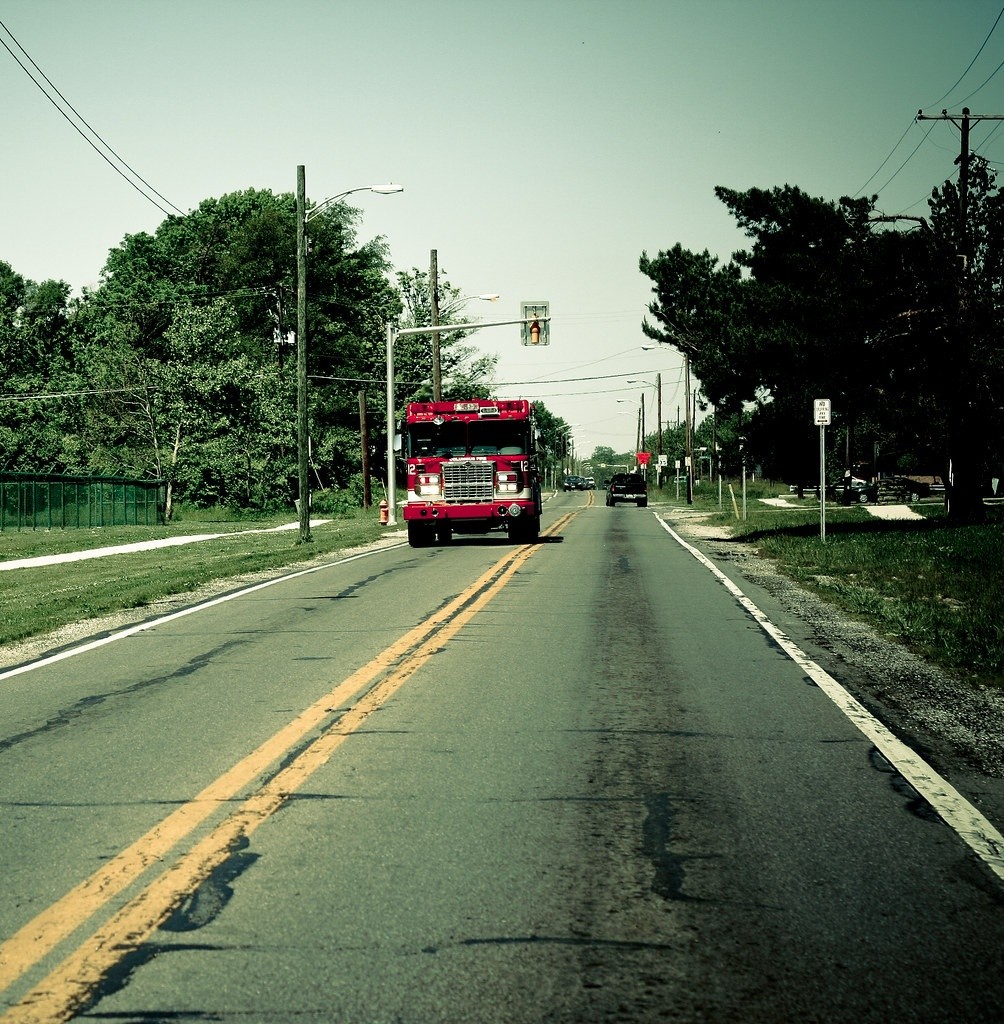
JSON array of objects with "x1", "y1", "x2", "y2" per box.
[{"x1": 377, "y1": 498, "x2": 389, "y2": 526}]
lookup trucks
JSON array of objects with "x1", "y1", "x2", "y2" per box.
[{"x1": 393, "y1": 396, "x2": 544, "y2": 549}]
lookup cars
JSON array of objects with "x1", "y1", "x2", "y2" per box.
[
  {"x1": 850, "y1": 476, "x2": 931, "y2": 504},
  {"x1": 562, "y1": 475, "x2": 584, "y2": 492},
  {"x1": 585, "y1": 477, "x2": 597, "y2": 490}
]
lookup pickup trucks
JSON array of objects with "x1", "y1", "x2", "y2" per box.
[{"x1": 604, "y1": 471, "x2": 648, "y2": 507}]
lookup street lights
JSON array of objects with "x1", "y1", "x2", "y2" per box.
[
  {"x1": 616, "y1": 407, "x2": 641, "y2": 467},
  {"x1": 627, "y1": 372, "x2": 663, "y2": 455},
  {"x1": 298, "y1": 162, "x2": 404, "y2": 540},
  {"x1": 430, "y1": 248, "x2": 500, "y2": 400},
  {"x1": 642, "y1": 341, "x2": 695, "y2": 506},
  {"x1": 616, "y1": 392, "x2": 644, "y2": 452}
]
